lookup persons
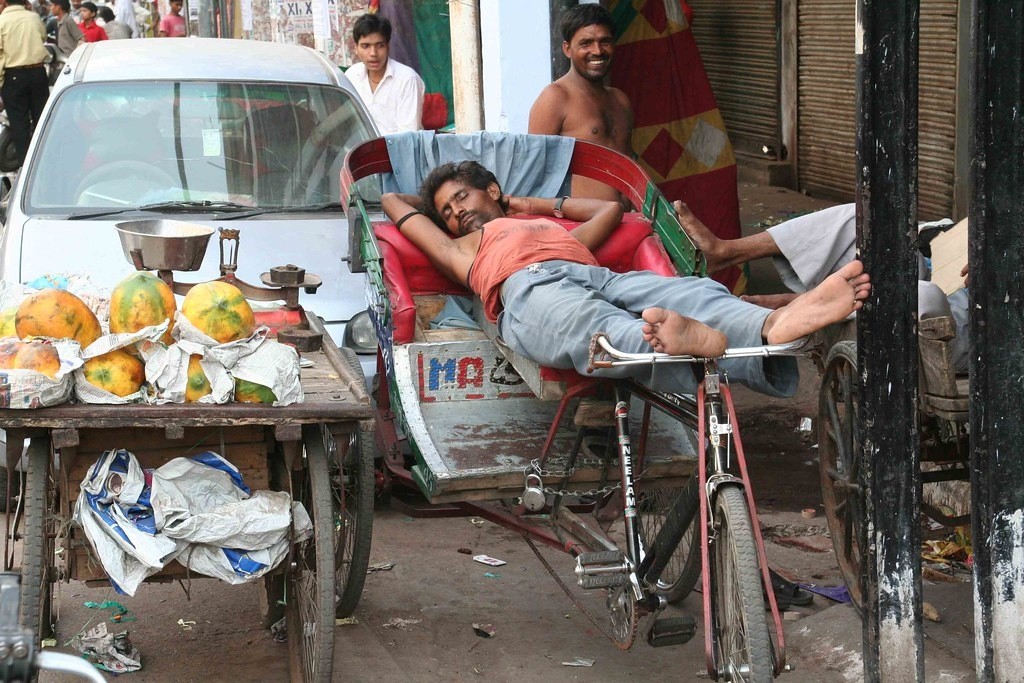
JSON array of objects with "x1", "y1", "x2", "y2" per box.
[
  {"x1": 527, "y1": 4, "x2": 635, "y2": 161},
  {"x1": 380, "y1": 161, "x2": 872, "y2": 397},
  {"x1": 342, "y1": 12, "x2": 424, "y2": 135},
  {"x1": 671, "y1": 199, "x2": 971, "y2": 379},
  {"x1": 0, "y1": 0, "x2": 191, "y2": 167}
]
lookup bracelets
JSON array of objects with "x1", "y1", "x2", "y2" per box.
[{"x1": 396, "y1": 211, "x2": 420, "y2": 230}]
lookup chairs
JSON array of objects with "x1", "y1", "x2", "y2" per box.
[
  {"x1": 239, "y1": 105, "x2": 324, "y2": 178},
  {"x1": 82, "y1": 116, "x2": 168, "y2": 168}
]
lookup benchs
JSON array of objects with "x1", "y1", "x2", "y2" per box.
[{"x1": 372, "y1": 212, "x2": 679, "y2": 420}]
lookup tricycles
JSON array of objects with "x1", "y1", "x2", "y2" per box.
[
  {"x1": 812, "y1": 315, "x2": 970, "y2": 619},
  {"x1": 0, "y1": 305, "x2": 378, "y2": 682},
  {"x1": 337, "y1": 127, "x2": 824, "y2": 683}
]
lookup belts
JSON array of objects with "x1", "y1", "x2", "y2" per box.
[{"x1": 9, "y1": 63, "x2": 43, "y2": 68}]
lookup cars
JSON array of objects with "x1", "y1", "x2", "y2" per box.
[{"x1": 0, "y1": 35, "x2": 410, "y2": 466}]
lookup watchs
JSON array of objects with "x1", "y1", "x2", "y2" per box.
[{"x1": 553, "y1": 195, "x2": 570, "y2": 219}]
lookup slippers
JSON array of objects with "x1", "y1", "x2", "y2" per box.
[{"x1": 760, "y1": 566, "x2": 813, "y2": 609}]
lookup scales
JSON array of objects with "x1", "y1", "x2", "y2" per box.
[{"x1": 116, "y1": 219, "x2": 324, "y2": 339}]
